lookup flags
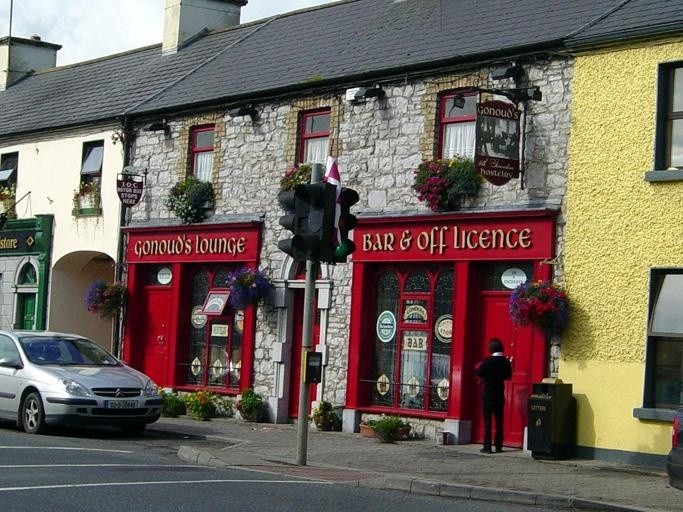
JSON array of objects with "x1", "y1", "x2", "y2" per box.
[{"x1": 324, "y1": 128, "x2": 343, "y2": 249}]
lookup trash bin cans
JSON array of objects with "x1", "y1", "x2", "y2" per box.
[{"x1": 528, "y1": 383, "x2": 577, "y2": 459}]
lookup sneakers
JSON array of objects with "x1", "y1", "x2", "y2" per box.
[{"x1": 480, "y1": 446, "x2": 504, "y2": 454}]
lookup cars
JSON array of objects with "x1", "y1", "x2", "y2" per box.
[
  {"x1": 0, "y1": 330, "x2": 163, "y2": 434},
  {"x1": 377, "y1": 349, "x2": 449, "y2": 393}
]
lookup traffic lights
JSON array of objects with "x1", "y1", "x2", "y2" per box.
[{"x1": 278, "y1": 183, "x2": 359, "y2": 263}]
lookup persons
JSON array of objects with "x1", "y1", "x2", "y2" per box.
[{"x1": 473, "y1": 336, "x2": 512, "y2": 454}]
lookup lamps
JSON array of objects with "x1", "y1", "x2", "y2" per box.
[
  {"x1": 492, "y1": 66, "x2": 525, "y2": 80},
  {"x1": 354, "y1": 87, "x2": 386, "y2": 99},
  {"x1": 142, "y1": 123, "x2": 170, "y2": 131},
  {"x1": 229, "y1": 107, "x2": 258, "y2": 117}
]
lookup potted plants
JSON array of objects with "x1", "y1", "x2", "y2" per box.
[{"x1": 162, "y1": 387, "x2": 411, "y2": 442}]
